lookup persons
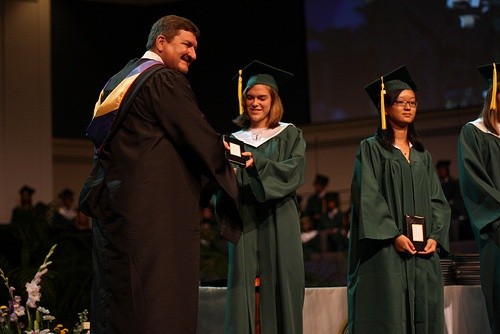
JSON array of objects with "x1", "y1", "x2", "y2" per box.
[
  {"x1": 457, "y1": 63, "x2": 500, "y2": 334},
  {"x1": 53, "y1": 189, "x2": 80, "y2": 229},
  {"x1": 11, "y1": 185, "x2": 35, "y2": 224},
  {"x1": 300, "y1": 175, "x2": 351, "y2": 254},
  {"x1": 78, "y1": 15, "x2": 242, "y2": 334},
  {"x1": 435, "y1": 159, "x2": 461, "y2": 207},
  {"x1": 347, "y1": 65, "x2": 451, "y2": 334},
  {"x1": 212, "y1": 61, "x2": 305, "y2": 334}
]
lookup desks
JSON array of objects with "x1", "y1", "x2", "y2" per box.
[{"x1": 197, "y1": 286, "x2": 491, "y2": 334}]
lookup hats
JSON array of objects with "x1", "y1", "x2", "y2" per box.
[
  {"x1": 19, "y1": 185, "x2": 36, "y2": 197},
  {"x1": 59, "y1": 188, "x2": 74, "y2": 198},
  {"x1": 478, "y1": 62, "x2": 500, "y2": 110},
  {"x1": 364, "y1": 65, "x2": 420, "y2": 130},
  {"x1": 324, "y1": 192, "x2": 338, "y2": 202},
  {"x1": 233, "y1": 59, "x2": 295, "y2": 116},
  {"x1": 314, "y1": 174, "x2": 328, "y2": 186},
  {"x1": 435, "y1": 159, "x2": 450, "y2": 169}
]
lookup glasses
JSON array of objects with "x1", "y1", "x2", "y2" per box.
[{"x1": 394, "y1": 99, "x2": 418, "y2": 107}]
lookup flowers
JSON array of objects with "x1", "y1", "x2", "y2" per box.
[{"x1": 0, "y1": 243, "x2": 90, "y2": 334}]
